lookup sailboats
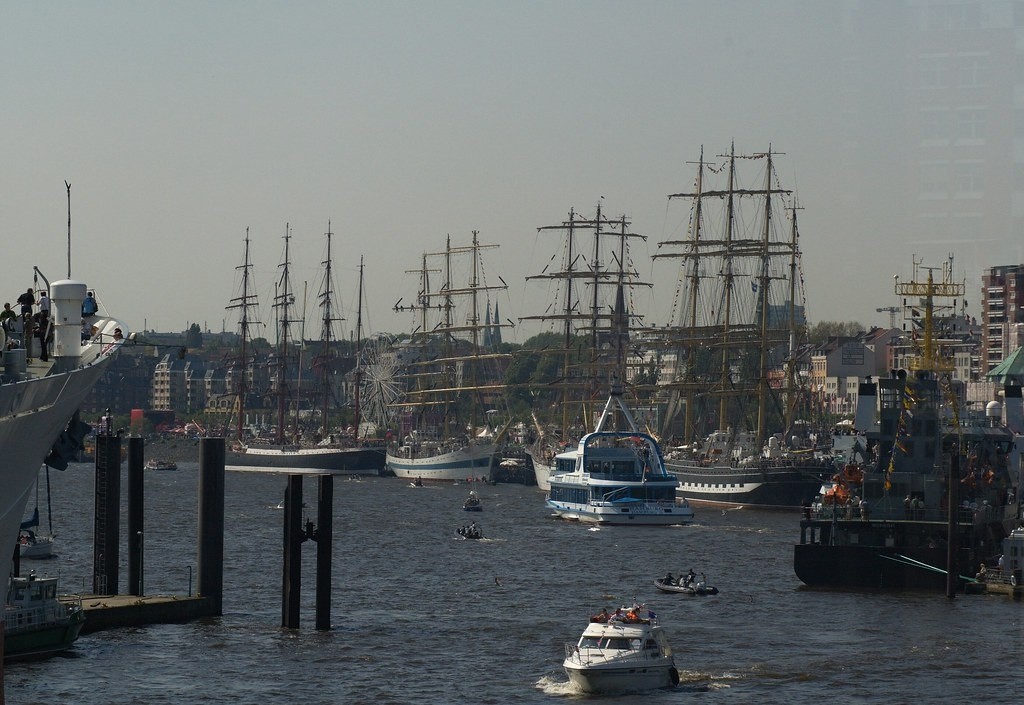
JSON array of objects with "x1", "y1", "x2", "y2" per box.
[{"x1": 206, "y1": 139, "x2": 1023, "y2": 608}]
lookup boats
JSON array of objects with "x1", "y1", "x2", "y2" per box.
[
  {"x1": 0, "y1": 266, "x2": 137, "y2": 603},
  {"x1": 145, "y1": 459, "x2": 177, "y2": 470},
  {"x1": 562, "y1": 606, "x2": 678, "y2": 694},
  {"x1": 0, "y1": 566, "x2": 88, "y2": 657},
  {"x1": 462, "y1": 497, "x2": 483, "y2": 511},
  {"x1": 458, "y1": 527, "x2": 480, "y2": 539},
  {"x1": 654, "y1": 577, "x2": 718, "y2": 594},
  {"x1": 544, "y1": 375, "x2": 696, "y2": 524}
]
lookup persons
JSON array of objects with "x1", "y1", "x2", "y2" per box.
[
  {"x1": 662, "y1": 568, "x2": 706, "y2": 587},
  {"x1": 0, "y1": 287, "x2": 123, "y2": 361},
  {"x1": 951, "y1": 496, "x2": 1024, "y2": 574},
  {"x1": 351, "y1": 473, "x2": 360, "y2": 479},
  {"x1": 466, "y1": 496, "x2": 480, "y2": 506},
  {"x1": 799, "y1": 491, "x2": 926, "y2": 520},
  {"x1": 595, "y1": 607, "x2": 640, "y2": 625},
  {"x1": 412, "y1": 476, "x2": 422, "y2": 486},
  {"x1": 460, "y1": 521, "x2": 479, "y2": 537}
]
